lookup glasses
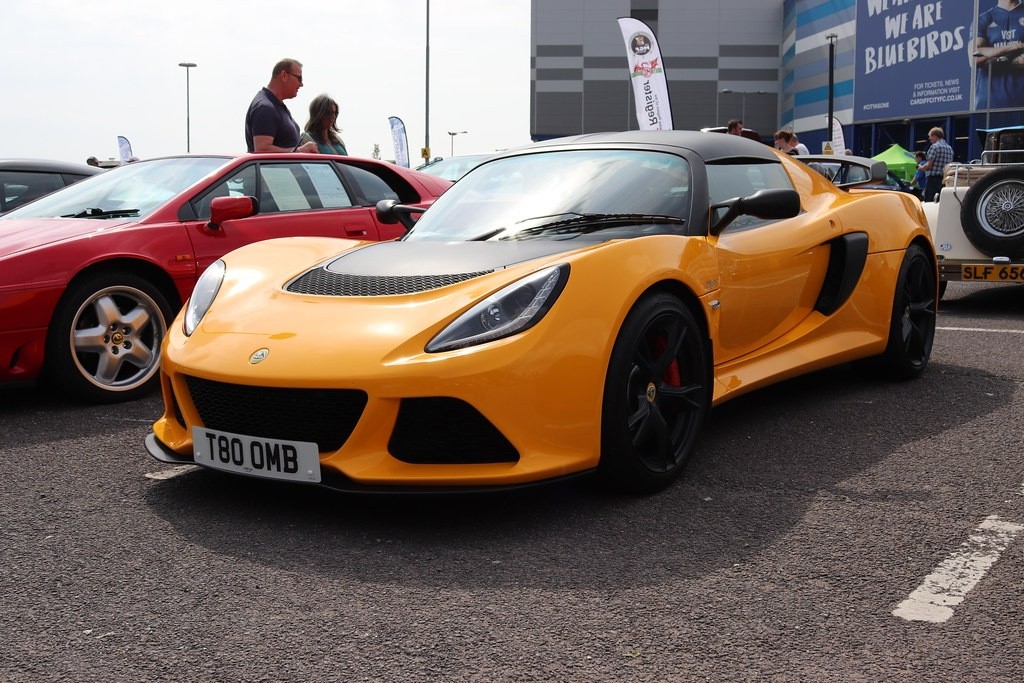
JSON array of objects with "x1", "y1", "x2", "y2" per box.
[{"x1": 287, "y1": 72, "x2": 302, "y2": 82}]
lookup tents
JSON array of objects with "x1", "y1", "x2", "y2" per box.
[{"x1": 871, "y1": 143, "x2": 917, "y2": 187}]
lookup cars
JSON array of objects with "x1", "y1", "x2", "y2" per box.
[
  {"x1": 791, "y1": 155, "x2": 921, "y2": 201},
  {"x1": 0, "y1": 153, "x2": 455, "y2": 408},
  {"x1": 921, "y1": 126, "x2": 1024, "y2": 300},
  {"x1": 0, "y1": 159, "x2": 109, "y2": 213}
]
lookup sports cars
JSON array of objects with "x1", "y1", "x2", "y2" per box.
[{"x1": 143, "y1": 129, "x2": 941, "y2": 501}]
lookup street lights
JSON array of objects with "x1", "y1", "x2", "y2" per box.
[
  {"x1": 448, "y1": 132, "x2": 457, "y2": 156},
  {"x1": 722, "y1": 89, "x2": 768, "y2": 128},
  {"x1": 973, "y1": 52, "x2": 1008, "y2": 130},
  {"x1": 179, "y1": 63, "x2": 197, "y2": 153}
]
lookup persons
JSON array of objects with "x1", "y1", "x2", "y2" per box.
[
  {"x1": 774, "y1": 130, "x2": 799, "y2": 155},
  {"x1": 790, "y1": 133, "x2": 810, "y2": 155},
  {"x1": 967, "y1": 0, "x2": 1024, "y2": 111},
  {"x1": 917, "y1": 126, "x2": 954, "y2": 202},
  {"x1": 909, "y1": 152, "x2": 927, "y2": 191},
  {"x1": 301, "y1": 95, "x2": 348, "y2": 156},
  {"x1": 245, "y1": 58, "x2": 319, "y2": 154},
  {"x1": 726, "y1": 119, "x2": 744, "y2": 136}
]
